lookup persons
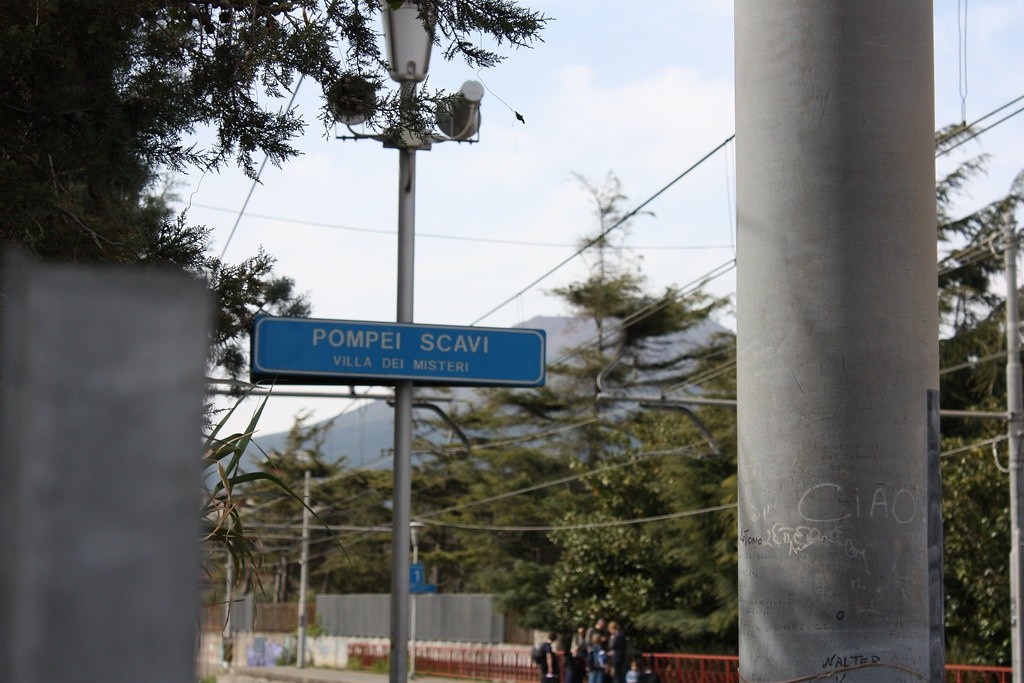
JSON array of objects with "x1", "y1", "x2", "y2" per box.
[
  {"x1": 564, "y1": 617, "x2": 659, "y2": 683},
  {"x1": 537, "y1": 632, "x2": 561, "y2": 683}
]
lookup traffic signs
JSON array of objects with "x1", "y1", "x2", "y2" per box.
[{"x1": 248, "y1": 315, "x2": 549, "y2": 389}]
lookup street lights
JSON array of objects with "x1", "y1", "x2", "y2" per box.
[{"x1": 326, "y1": 0, "x2": 484, "y2": 683}]
